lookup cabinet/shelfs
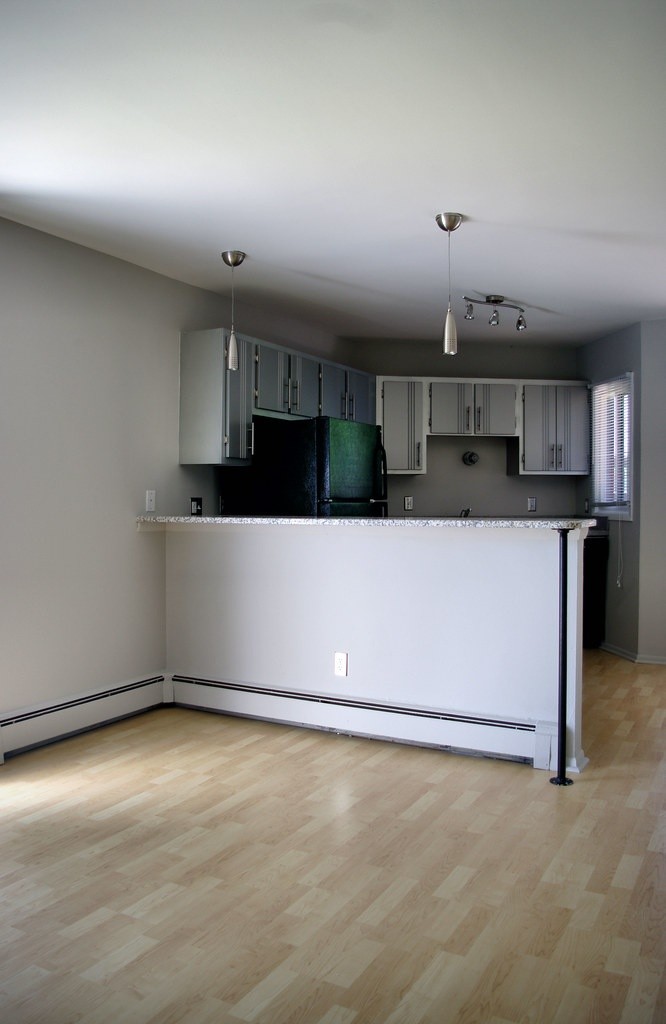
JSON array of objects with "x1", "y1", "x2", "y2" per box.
[
  {"x1": 179, "y1": 328, "x2": 376, "y2": 467},
  {"x1": 378, "y1": 376, "x2": 592, "y2": 477}
]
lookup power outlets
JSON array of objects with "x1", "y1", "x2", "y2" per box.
[{"x1": 334, "y1": 651, "x2": 348, "y2": 676}]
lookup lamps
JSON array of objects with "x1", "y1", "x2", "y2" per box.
[
  {"x1": 463, "y1": 294, "x2": 526, "y2": 332},
  {"x1": 220, "y1": 250, "x2": 246, "y2": 370},
  {"x1": 435, "y1": 213, "x2": 464, "y2": 355}
]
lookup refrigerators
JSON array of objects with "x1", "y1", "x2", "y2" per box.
[{"x1": 217, "y1": 415, "x2": 388, "y2": 518}]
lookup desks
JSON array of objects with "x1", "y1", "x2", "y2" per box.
[{"x1": 133, "y1": 514, "x2": 597, "y2": 775}]
